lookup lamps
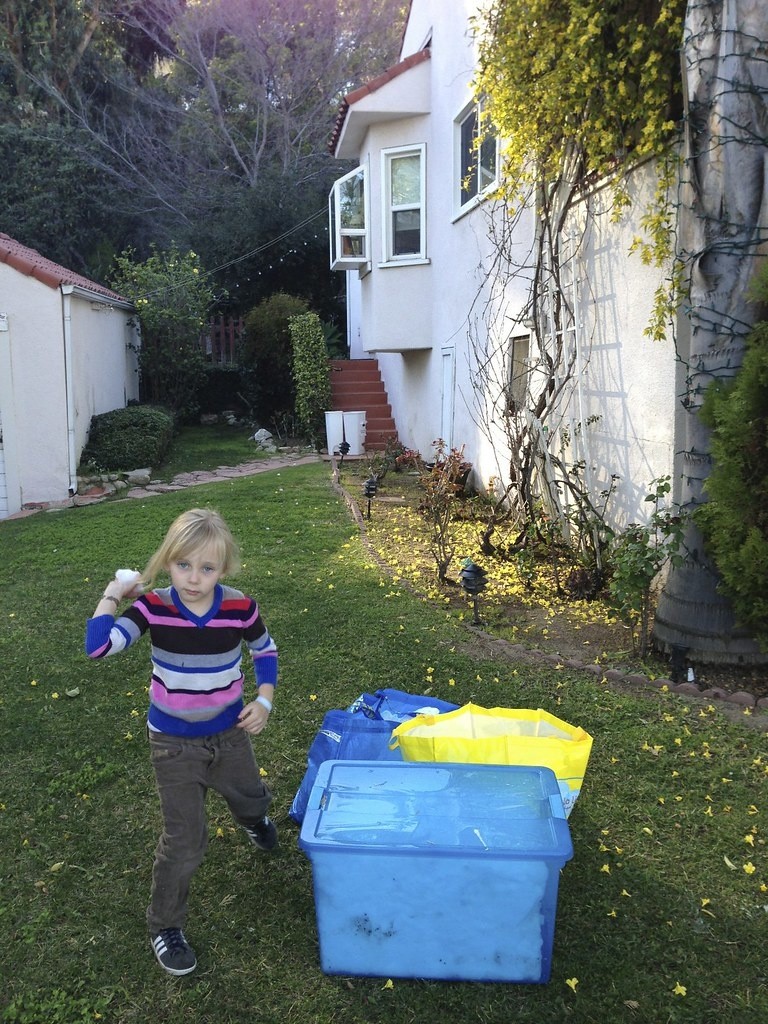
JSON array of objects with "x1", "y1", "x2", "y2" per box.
[
  {"x1": 339, "y1": 441, "x2": 351, "y2": 464},
  {"x1": 462, "y1": 562, "x2": 490, "y2": 624},
  {"x1": 361, "y1": 478, "x2": 379, "y2": 521}
]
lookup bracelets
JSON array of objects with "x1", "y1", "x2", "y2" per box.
[
  {"x1": 254, "y1": 695, "x2": 272, "y2": 713},
  {"x1": 99, "y1": 595, "x2": 119, "y2": 606}
]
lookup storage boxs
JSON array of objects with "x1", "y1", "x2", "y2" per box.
[{"x1": 297, "y1": 759, "x2": 574, "y2": 986}]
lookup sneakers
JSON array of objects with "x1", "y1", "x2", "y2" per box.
[
  {"x1": 150, "y1": 924, "x2": 199, "y2": 975},
  {"x1": 247, "y1": 813, "x2": 278, "y2": 851}
]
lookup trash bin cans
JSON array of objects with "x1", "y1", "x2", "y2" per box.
[
  {"x1": 341, "y1": 410, "x2": 367, "y2": 456},
  {"x1": 323, "y1": 410, "x2": 344, "y2": 455}
]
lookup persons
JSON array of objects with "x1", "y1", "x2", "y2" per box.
[{"x1": 85, "y1": 509, "x2": 279, "y2": 975}]
